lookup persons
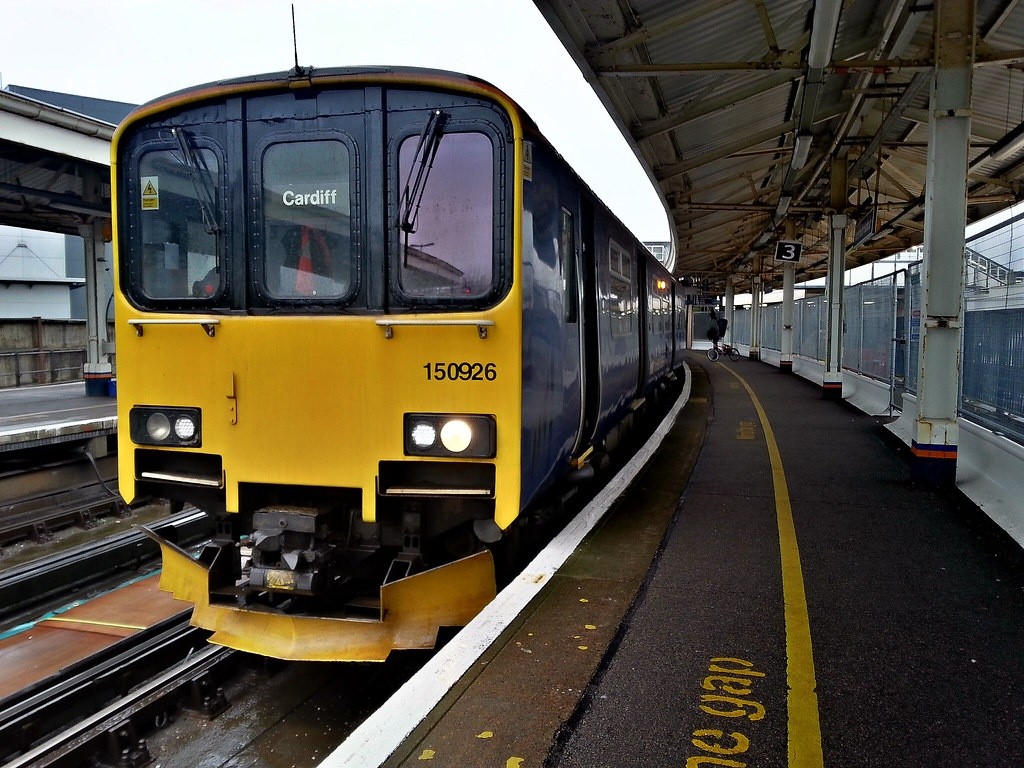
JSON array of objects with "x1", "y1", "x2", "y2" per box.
[{"x1": 708, "y1": 311, "x2": 720, "y2": 359}]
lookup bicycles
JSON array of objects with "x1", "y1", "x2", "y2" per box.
[{"x1": 706, "y1": 338, "x2": 740, "y2": 363}]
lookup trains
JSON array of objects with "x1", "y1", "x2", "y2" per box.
[{"x1": 110, "y1": 63, "x2": 687, "y2": 663}]
